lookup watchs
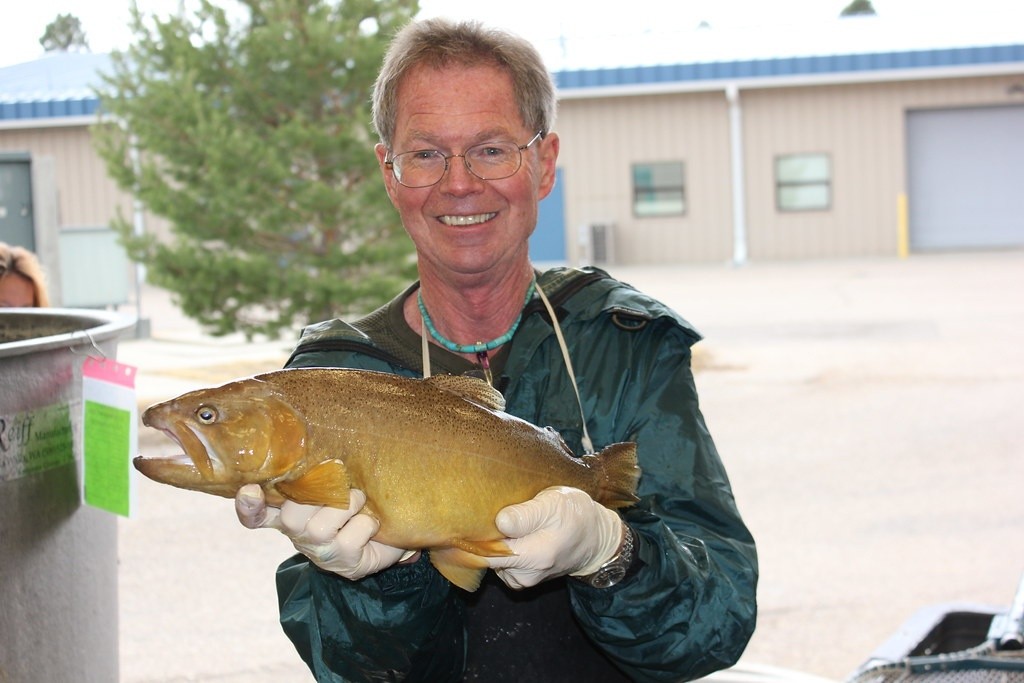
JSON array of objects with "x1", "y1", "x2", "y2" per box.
[{"x1": 575, "y1": 518, "x2": 634, "y2": 587}]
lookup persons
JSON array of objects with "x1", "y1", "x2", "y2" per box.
[
  {"x1": 231, "y1": 18, "x2": 760, "y2": 683},
  {"x1": 0, "y1": 241, "x2": 49, "y2": 307}
]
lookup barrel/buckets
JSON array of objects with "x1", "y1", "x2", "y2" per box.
[{"x1": 0, "y1": 304, "x2": 138, "y2": 683}]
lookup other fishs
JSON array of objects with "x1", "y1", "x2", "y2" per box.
[{"x1": 132, "y1": 367, "x2": 642, "y2": 593}]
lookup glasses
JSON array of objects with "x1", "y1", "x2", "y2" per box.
[{"x1": 384, "y1": 130, "x2": 542, "y2": 188}]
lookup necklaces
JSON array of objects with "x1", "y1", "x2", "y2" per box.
[{"x1": 419, "y1": 272, "x2": 537, "y2": 352}]
